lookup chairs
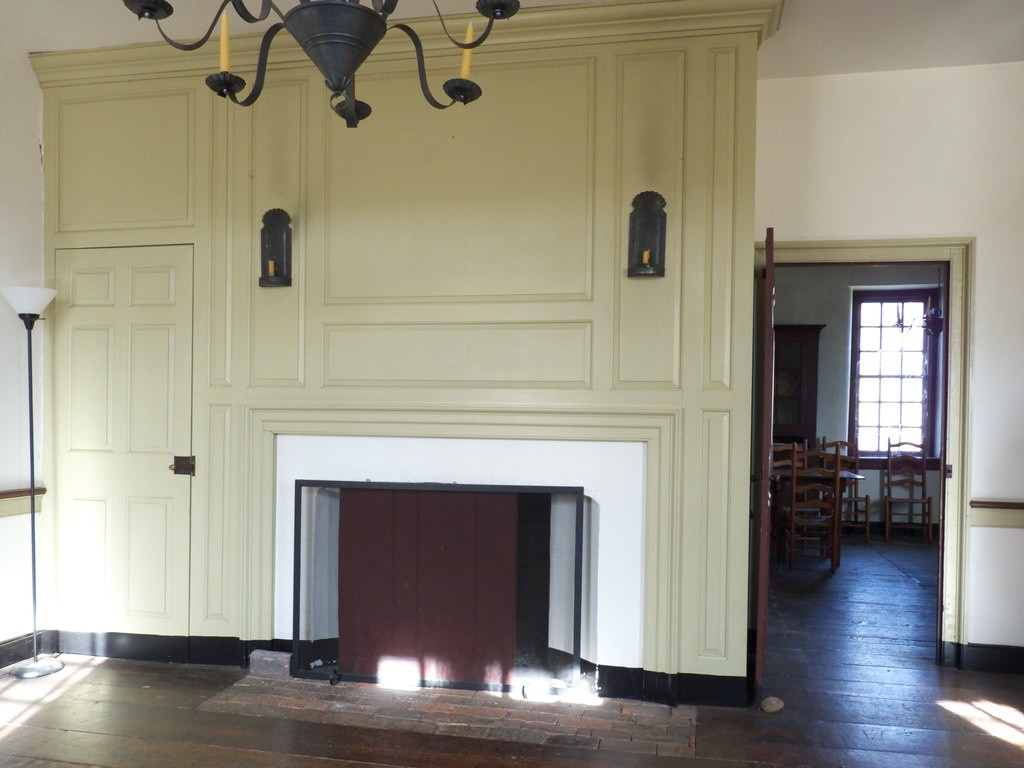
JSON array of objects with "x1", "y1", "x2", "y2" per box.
[
  {"x1": 771, "y1": 436, "x2": 870, "y2": 575},
  {"x1": 884, "y1": 437, "x2": 932, "y2": 544}
]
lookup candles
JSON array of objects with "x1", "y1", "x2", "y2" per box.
[
  {"x1": 269, "y1": 259, "x2": 274, "y2": 277},
  {"x1": 643, "y1": 249, "x2": 649, "y2": 264},
  {"x1": 220, "y1": 11, "x2": 230, "y2": 74},
  {"x1": 460, "y1": 21, "x2": 473, "y2": 80}
]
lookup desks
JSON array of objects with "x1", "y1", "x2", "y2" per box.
[{"x1": 772, "y1": 469, "x2": 866, "y2": 555}]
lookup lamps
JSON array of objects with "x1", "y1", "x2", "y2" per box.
[
  {"x1": 0, "y1": 288, "x2": 65, "y2": 679},
  {"x1": 922, "y1": 309, "x2": 944, "y2": 340},
  {"x1": 121, "y1": 0, "x2": 522, "y2": 130}
]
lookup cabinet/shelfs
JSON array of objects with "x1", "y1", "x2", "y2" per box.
[{"x1": 774, "y1": 324, "x2": 830, "y2": 531}]
